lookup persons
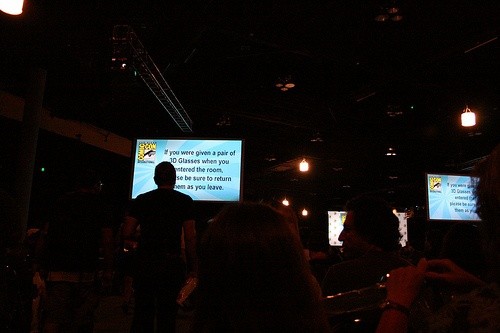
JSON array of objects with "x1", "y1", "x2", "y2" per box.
[
  {"x1": 309, "y1": 232, "x2": 333, "y2": 274},
  {"x1": 402, "y1": 241, "x2": 415, "y2": 256},
  {"x1": 436, "y1": 222, "x2": 487, "y2": 292},
  {"x1": 190, "y1": 201, "x2": 428, "y2": 333},
  {"x1": 423, "y1": 230, "x2": 446, "y2": 258},
  {"x1": 422, "y1": 146, "x2": 500, "y2": 333},
  {"x1": 122, "y1": 163, "x2": 200, "y2": 333},
  {"x1": 31, "y1": 170, "x2": 115, "y2": 333},
  {"x1": 320, "y1": 196, "x2": 412, "y2": 311},
  {"x1": 94, "y1": 220, "x2": 140, "y2": 263}
]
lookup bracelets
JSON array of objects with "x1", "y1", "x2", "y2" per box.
[{"x1": 382, "y1": 303, "x2": 412, "y2": 317}]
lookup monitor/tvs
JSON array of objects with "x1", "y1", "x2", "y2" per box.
[
  {"x1": 130, "y1": 137, "x2": 247, "y2": 205},
  {"x1": 424, "y1": 172, "x2": 483, "y2": 223}
]
